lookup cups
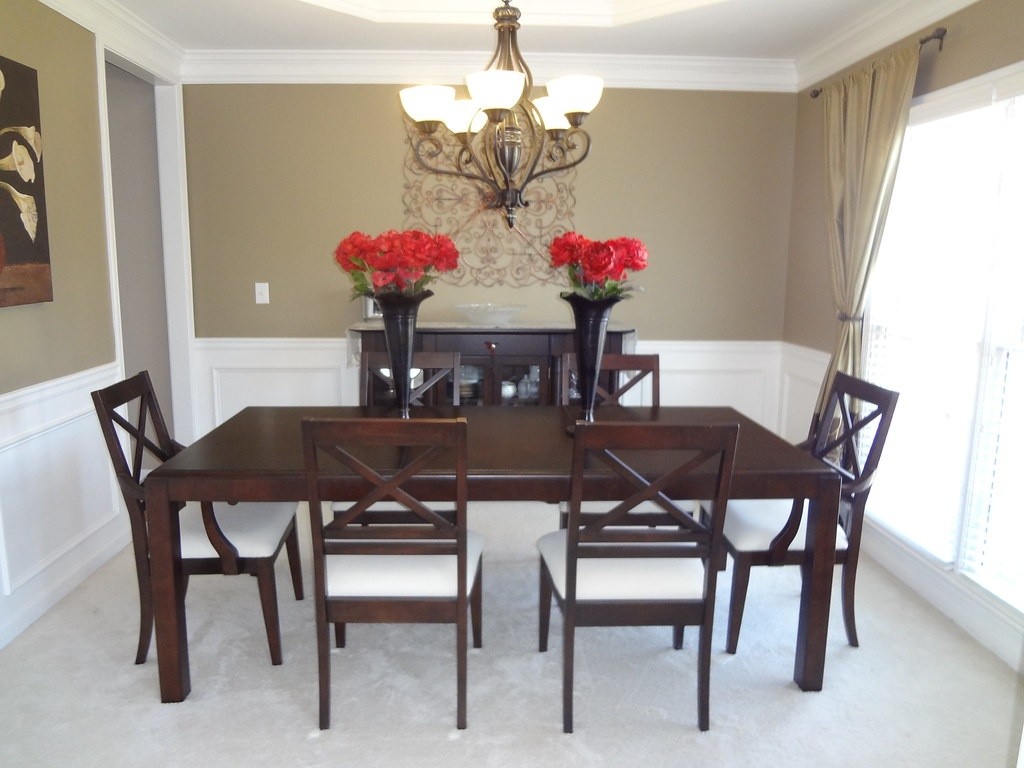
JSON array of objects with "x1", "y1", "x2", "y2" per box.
[{"x1": 518, "y1": 375, "x2": 531, "y2": 399}]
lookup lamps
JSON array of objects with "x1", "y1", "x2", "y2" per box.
[{"x1": 399, "y1": 0, "x2": 606, "y2": 228}]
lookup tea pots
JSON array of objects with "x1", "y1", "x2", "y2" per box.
[{"x1": 502, "y1": 381, "x2": 517, "y2": 398}]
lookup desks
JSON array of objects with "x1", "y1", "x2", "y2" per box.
[{"x1": 140, "y1": 405, "x2": 838, "y2": 700}]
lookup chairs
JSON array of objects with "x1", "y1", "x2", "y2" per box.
[
  {"x1": 558, "y1": 353, "x2": 695, "y2": 535},
  {"x1": 300, "y1": 415, "x2": 484, "y2": 732},
  {"x1": 330, "y1": 349, "x2": 461, "y2": 524},
  {"x1": 93, "y1": 368, "x2": 305, "y2": 668},
  {"x1": 699, "y1": 371, "x2": 899, "y2": 656}
]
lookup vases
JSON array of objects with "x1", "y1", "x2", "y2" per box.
[
  {"x1": 368, "y1": 291, "x2": 435, "y2": 418},
  {"x1": 559, "y1": 290, "x2": 624, "y2": 438}
]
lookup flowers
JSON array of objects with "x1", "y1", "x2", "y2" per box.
[
  {"x1": 550, "y1": 232, "x2": 650, "y2": 302},
  {"x1": 338, "y1": 230, "x2": 463, "y2": 301}
]
